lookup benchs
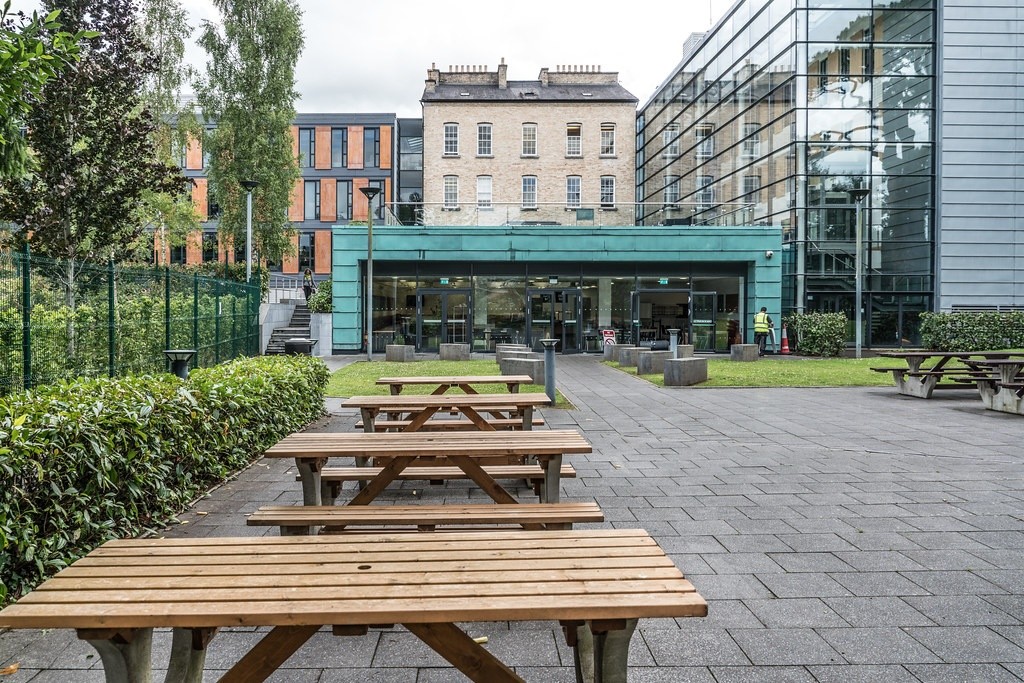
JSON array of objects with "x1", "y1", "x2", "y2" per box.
[
  {"x1": 245, "y1": 501, "x2": 605, "y2": 533},
  {"x1": 295, "y1": 464, "x2": 577, "y2": 504},
  {"x1": 354, "y1": 418, "x2": 546, "y2": 431}
]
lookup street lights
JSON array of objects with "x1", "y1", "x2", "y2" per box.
[
  {"x1": 238, "y1": 180, "x2": 259, "y2": 285},
  {"x1": 358, "y1": 187, "x2": 382, "y2": 361}
]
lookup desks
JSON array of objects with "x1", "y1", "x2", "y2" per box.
[
  {"x1": 264, "y1": 428, "x2": 593, "y2": 503},
  {"x1": 341, "y1": 393, "x2": 552, "y2": 431},
  {"x1": 375, "y1": 375, "x2": 534, "y2": 393},
  {"x1": 1, "y1": 529, "x2": 711, "y2": 683}
]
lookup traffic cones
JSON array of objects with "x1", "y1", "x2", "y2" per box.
[{"x1": 777, "y1": 324, "x2": 793, "y2": 354}]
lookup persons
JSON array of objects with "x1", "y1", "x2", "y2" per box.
[
  {"x1": 726, "y1": 307, "x2": 740, "y2": 351},
  {"x1": 301, "y1": 268, "x2": 316, "y2": 305},
  {"x1": 752, "y1": 307, "x2": 774, "y2": 358}
]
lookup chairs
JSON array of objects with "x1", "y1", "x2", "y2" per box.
[{"x1": 868, "y1": 350, "x2": 1024, "y2": 416}]
